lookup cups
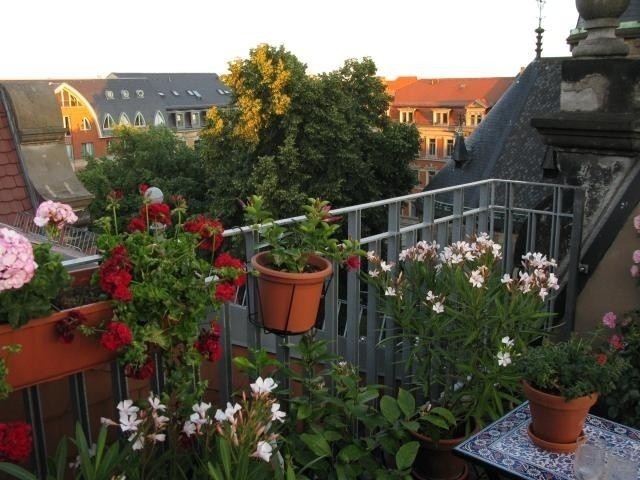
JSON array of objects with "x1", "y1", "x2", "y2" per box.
[{"x1": 574, "y1": 435, "x2": 640, "y2": 480}]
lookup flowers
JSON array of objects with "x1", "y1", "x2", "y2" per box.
[{"x1": 0, "y1": 183, "x2": 640, "y2": 480}]
